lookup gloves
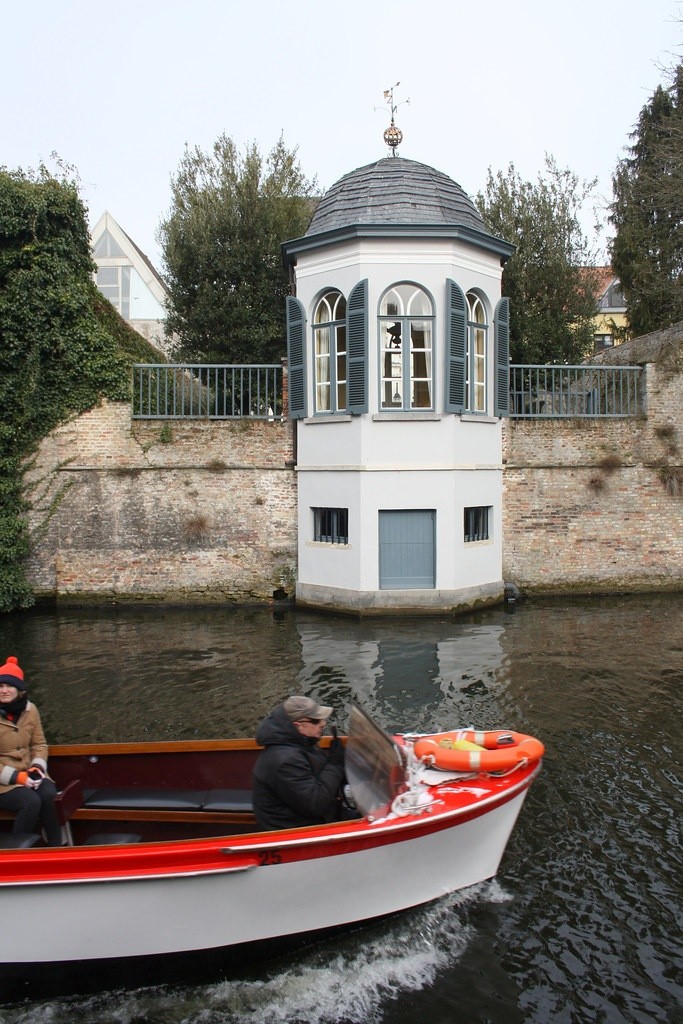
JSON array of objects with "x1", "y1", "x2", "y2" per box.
[
  {"x1": 331, "y1": 738, "x2": 344, "y2": 767},
  {"x1": 359, "y1": 764, "x2": 375, "y2": 780}
]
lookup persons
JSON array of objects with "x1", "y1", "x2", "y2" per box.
[
  {"x1": 0, "y1": 663, "x2": 63, "y2": 847},
  {"x1": 250, "y1": 695, "x2": 364, "y2": 831}
]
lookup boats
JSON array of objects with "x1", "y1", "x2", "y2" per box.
[{"x1": 0, "y1": 726, "x2": 544, "y2": 963}]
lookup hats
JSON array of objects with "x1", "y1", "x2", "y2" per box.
[
  {"x1": 284, "y1": 696, "x2": 333, "y2": 722},
  {"x1": 0, "y1": 655, "x2": 24, "y2": 692}
]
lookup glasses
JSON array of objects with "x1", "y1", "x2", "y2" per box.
[{"x1": 297, "y1": 718, "x2": 321, "y2": 725}]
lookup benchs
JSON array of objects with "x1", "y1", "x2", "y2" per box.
[{"x1": 1, "y1": 770, "x2": 257, "y2": 849}]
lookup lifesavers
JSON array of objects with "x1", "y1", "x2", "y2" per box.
[{"x1": 413, "y1": 730, "x2": 545, "y2": 773}]
